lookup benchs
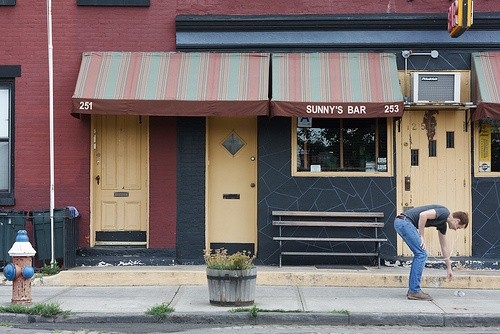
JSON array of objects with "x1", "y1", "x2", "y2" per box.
[{"x1": 272, "y1": 211, "x2": 386, "y2": 269}]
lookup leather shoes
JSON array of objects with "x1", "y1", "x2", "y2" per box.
[{"x1": 407, "y1": 288, "x2": 432, "y2": 302}]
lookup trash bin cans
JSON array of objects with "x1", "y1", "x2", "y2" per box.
[
  {"x1": 0, "y1": 210, "x2": 28, "y2": 272},
  {"x1": 30, "y1": 207, "x2": 76, "y2": 270}
]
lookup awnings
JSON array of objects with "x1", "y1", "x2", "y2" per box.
[
  {"x1": 71, "y1": 52, "x2": 270, "y2": 119},
  {"x1": 471, "y1": 51, "x2": 500, "y2": 123},
  {"x1": 271, "y1": 53, "x2": 404, "y2": 117}
]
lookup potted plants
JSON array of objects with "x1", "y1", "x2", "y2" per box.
[{"x1": 203, "y1": 247, "x2": 256, "y2": 306}]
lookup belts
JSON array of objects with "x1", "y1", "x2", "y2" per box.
[{"x1": 395, "y1": 212, "x2": 416, "y2": 226}]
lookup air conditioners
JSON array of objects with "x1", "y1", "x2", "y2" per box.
[{"x1": 411, "y1": 70, "x2": 461, "y2": 104}]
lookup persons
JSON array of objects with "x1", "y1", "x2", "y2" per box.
[{"x1": 394, "y1": 205, "x2": 468, "y2": 300}]
